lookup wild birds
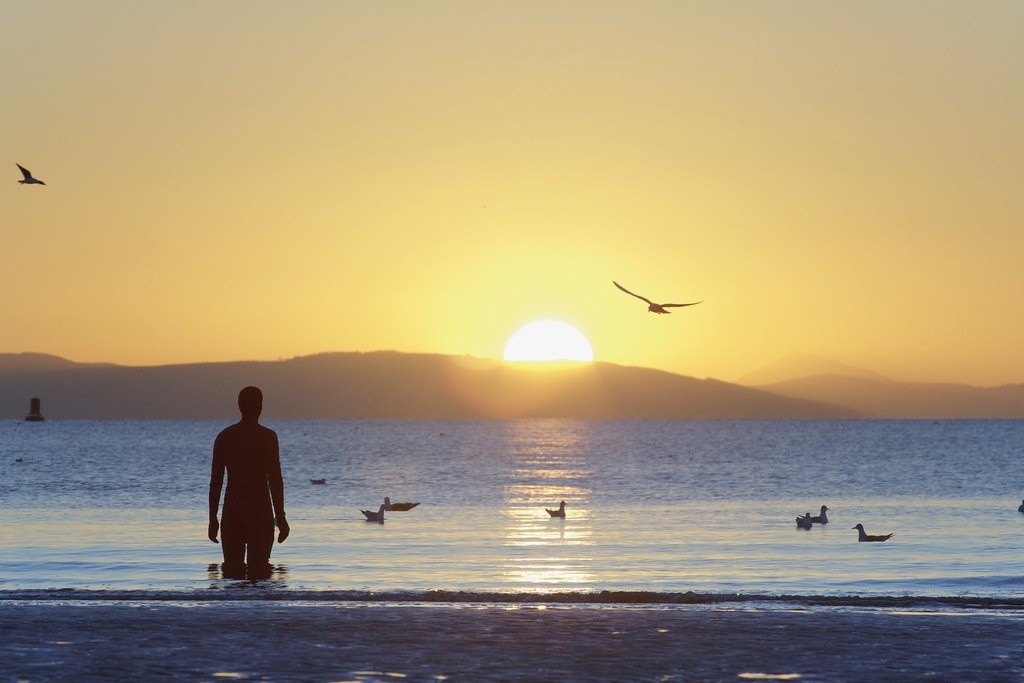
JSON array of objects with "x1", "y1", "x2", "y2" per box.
[
  {"x1": 852, "y1": 523, "x2": 895, "y2": 542},
  {"x1": 15, "y1": 163, "x2": 45, "y2": 186},
  {"x1": 544, "y1": 501, "x2": 567, "y2": 517},
  {"x1": 795, "y1": 505, "x2": 830, "y2": 529},
  {"x1": 309, "y1": 478, "x2": 326, "y2": 485},
  {"x1": 361, "y1": 496, "x2": 420, "y2": 520},
  {"x1": 613, "y1": 280, "x2": 703, "y2": 314}
]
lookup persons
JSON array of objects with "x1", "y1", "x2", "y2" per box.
[{"x1": 208, "y1": 386, "x2": 290, "y2": 565}]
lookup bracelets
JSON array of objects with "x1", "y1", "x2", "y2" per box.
[{"x1": 276, "y1": 512, "x2": 285, "y2": 517}]
